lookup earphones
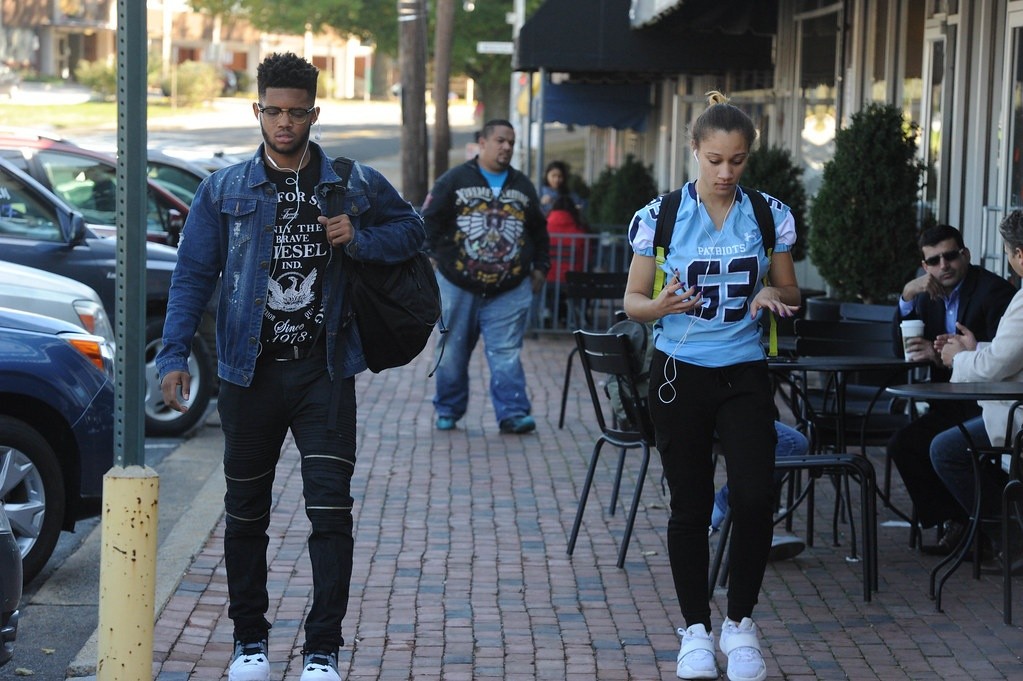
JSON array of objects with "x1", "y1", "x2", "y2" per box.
[
  {"x1": 694, "y1": 149, "x2": 699, "y2": 162},
  {"x1": 258, "y1": 113, "x2": 262, "y2": 127},
  {"x1": 315, "y1": 125, "x2": 321, "y2": 140}
]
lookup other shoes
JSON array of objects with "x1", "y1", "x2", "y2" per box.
[
  {"x1": 993, "y1": 530, "x2": 1022, "y2": 571},
  {"x1": 770, "y1": 535, "x2": 806, "y2": 562},
  {"x1": 543, "y1": 307, "x2": 552, "y2": 328}
]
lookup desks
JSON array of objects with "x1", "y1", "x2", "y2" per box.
[
  {"x1": 884, "y1": 381, "x2": 1023, "y2": 624},
  {"x1": 766, "y1": 357, "x2": 935, "y2": 555}
]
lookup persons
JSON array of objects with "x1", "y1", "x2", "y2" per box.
[
  {"x1": 888, "y1": 211, "x2": 1023, "y2": 576},
  {"x1": 707, "y1": 418, "x2": 808, "y2": 560},
  {"x1": 537, "y1": 161, "x2": 595, "y2": 329},
  {"x1": 420, "y1": 117, "x2": 537, "y2": 434},
  {"x1": 153, "y1": 51, "x2": 429, "y2": 681},
  {"x1": 623, "y1": 89, "x2": 802, "y2": 681}
]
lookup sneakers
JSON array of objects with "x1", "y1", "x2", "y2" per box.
[
  {"x1": 500, "y1": 415, "x2": 535, "y2": 433},
  {"x1": 934, "y1": 518, "x2": 965, "y2": 552},
  {"x1": 227, "y1": 636, "x2": 270, "y2": 681},
  {"x1": 300, "y1": 648, "x2": 341, "y2": 681},
  {"x1": 962, "y1": 532, "x2": 991, "y2": 561},
  {"x1": 719, "y1": 616, "x2": 767, "y2": 681},
  {"x1": 677, "y1": 623, "x2": 718, "y2": 679},
  {"x1": 436, "y1": 415, "x2": 456, "y2": 430}
]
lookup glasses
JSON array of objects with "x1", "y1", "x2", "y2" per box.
[
  {"x1": 258, "y1": 104, "x2": 314, "y2": 125},
  {"x1": 925, "y1": 249, "x2": 963, "y2": 266}
]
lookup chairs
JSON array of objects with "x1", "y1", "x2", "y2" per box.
[{"x1": 557, "y1": 270, "x2": 1023, "y2": 624}]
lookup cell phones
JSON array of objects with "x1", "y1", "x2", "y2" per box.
[{"x1": 669, "y1": 266, "x2": 692, "y2": 302}]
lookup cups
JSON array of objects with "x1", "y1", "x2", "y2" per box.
[{"x1": 899, "y1": 320, "x2": 925, "y2": 362}]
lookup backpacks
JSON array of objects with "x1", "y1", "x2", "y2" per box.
[{"x1": 602, "y1": 307, "x2": 653, "y2": 433}]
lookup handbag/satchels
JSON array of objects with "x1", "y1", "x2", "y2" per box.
[{"x1": 322, "y1": 156, "x2": 441, "y2": 374}]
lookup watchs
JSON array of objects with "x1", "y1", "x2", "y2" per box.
[{"x1": 951, "y1": 349, "x2": 963, "y2": 368}]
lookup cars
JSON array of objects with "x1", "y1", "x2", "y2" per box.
[
  {"x1": 1, "y1": 135, "x2": 233, "y2": 250},
  {"x1": 0, "y1": 261, "x2": 115, "y2": 664},
  {"x1": 0, "y1": 156, "x2": 223, "y2": 433}
]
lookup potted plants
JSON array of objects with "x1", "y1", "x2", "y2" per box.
[{"x1": 804, "y1": 98, "x2": 930, "y2": 388}]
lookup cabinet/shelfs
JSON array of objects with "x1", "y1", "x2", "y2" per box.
[{"x1": 760, "y1": 337, "x2": 847, "y2": 525}]
